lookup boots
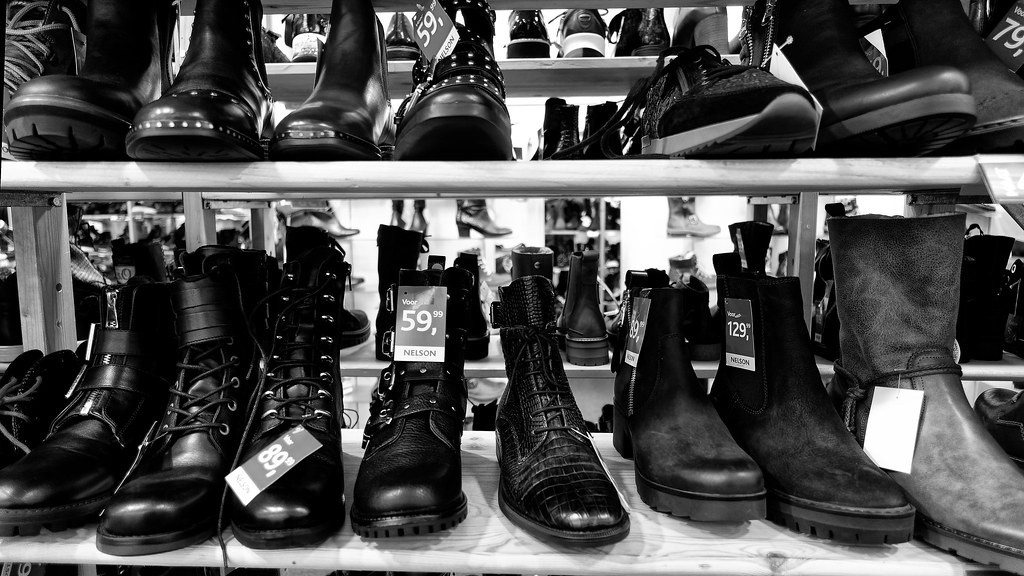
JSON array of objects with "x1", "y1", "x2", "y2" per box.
[{"x1": 0, "y1": 0, "x2": 1024, "y2": 576}]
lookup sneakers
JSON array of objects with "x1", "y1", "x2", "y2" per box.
[{"x1": 541, "y1": 44, "x2": 819, "y2": 160}]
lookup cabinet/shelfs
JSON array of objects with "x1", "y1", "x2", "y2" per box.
[{"x1": 0, "y1": 53, "x2": 1024, "y2": 576}]
